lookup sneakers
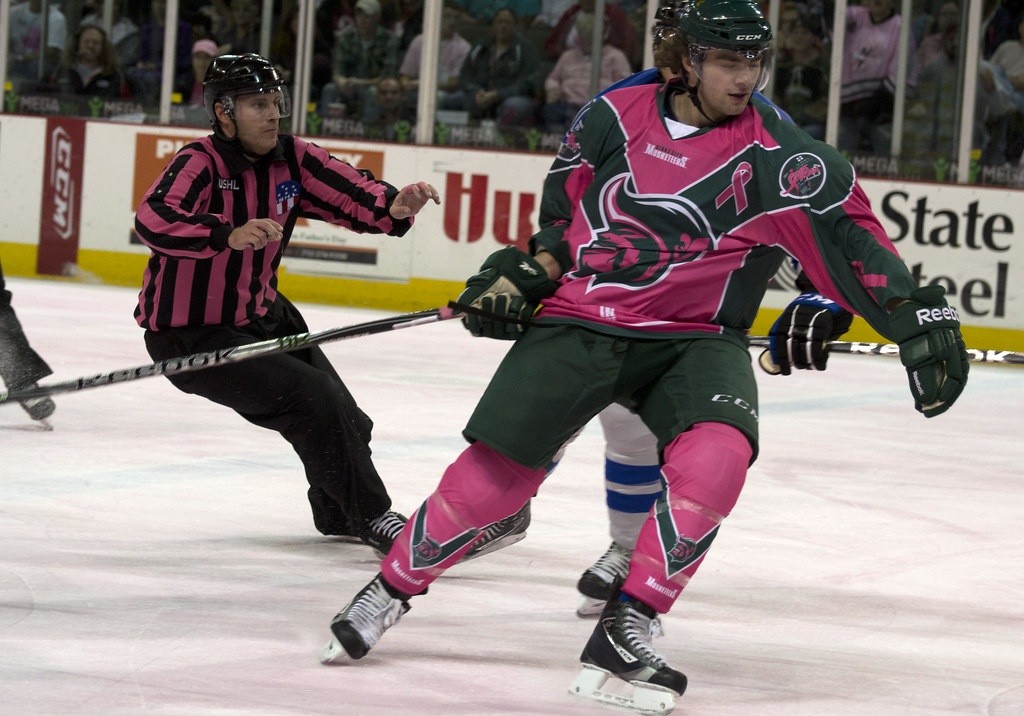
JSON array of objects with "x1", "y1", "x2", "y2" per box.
[
  {"x1": 307, "y1": 489, "x2": 408, "y2": 560},
  {"x1": 569, "y1": 573, "x2": 688, "y2": 716},
  {"x1": 322, "y1": 571, "x2": 429, "y2": 663},
  {"x1": 17, "y1": 382, "x2": 55, "y2": 431},
  {"x1": 577, "y1": 542, "x2": 633, "y2": 620}
]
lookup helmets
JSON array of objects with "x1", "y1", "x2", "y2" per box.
[
  {"x1": 202, "y1": 53, "x2": 291, "y2": 122},
  {"x1": 650, "y1": 0, "x2": 773, "y2": 93}
]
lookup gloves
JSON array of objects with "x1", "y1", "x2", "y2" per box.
[
  {"x1": 451, "y1": 246, "x2": 550, "y2": 341},
  {"x1": 888, "y1": 286, "x2": 970, "y2": 418},
  {"x1": 759, "y1": 292, "x2": 853, "y2": 377}
]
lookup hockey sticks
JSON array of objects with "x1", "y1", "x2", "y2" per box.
[
  {"x1": 0, "y1": 303, "x2": 464, "y2": 413},
  {"x1": 748, "y1": 336, "x2": 1022, "y2": 367}
]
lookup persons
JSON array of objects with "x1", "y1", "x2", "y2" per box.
[
  {"x1": 329, "y1": 0, "x2": 969, "y2": 696},
  {"x1": 0, "y1": 261, "x2": 55, "y2": 421},
  {"x1": 0, "y1": 0, "x2": 1024, "y2": 190},
  {"x1": 133, "y1": 51, "x2": 443, "y2": 558}
]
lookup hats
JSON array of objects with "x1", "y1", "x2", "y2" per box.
[
  {"x1": 355, "y1": 0, "x2": 381, "y2": 16},
  {"x1": 791, "y1": 17, "x2": 821, "y2": 36}
]
